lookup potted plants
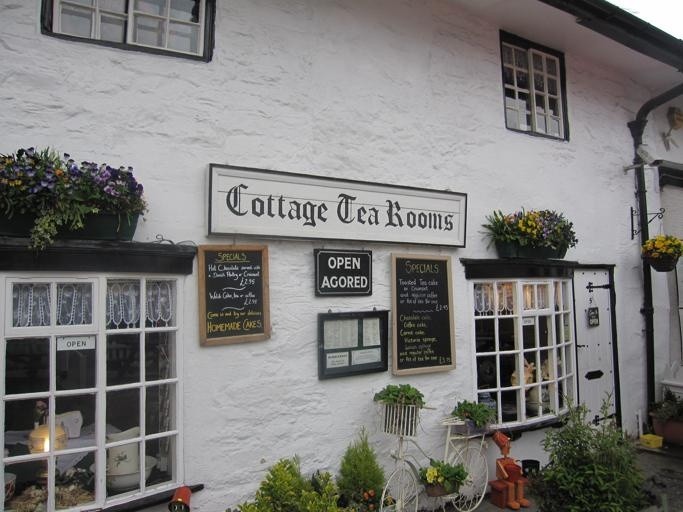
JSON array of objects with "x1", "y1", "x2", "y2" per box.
[
  {"x1": 647, "y1": 386, "x2": 681, "y2": 446},
  {"x1": 451, "y1": 401, "x2": 491, "y2": 435},
  {"x1": 373, "y1": 382, "x2": 423, "y2": 433}
]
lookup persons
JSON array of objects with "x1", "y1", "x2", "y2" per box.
[{"x1": 491, "y1": 430, "x2": 530, "y2": 510}]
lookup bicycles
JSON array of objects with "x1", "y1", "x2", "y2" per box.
[{"x1": 378, "y1": 402, "x2": 488, "y2": 512}]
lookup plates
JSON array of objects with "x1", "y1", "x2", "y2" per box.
[{"x1": 502, "y1": 408, "x2": 516, "y2": 414}]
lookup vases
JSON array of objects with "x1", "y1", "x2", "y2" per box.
[
  {"x1": 649, "y1": 251, "x2": 681, "y2": 274},
  {"x1": 423, "y1": 480, "x2": 462, "y2": 499}
]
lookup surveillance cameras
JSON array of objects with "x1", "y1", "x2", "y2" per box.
[{"x1": 635, "y1": 144, "x2": 665, "y2": 167}]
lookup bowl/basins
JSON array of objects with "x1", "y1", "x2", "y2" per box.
[
  {"x1": 3, "y1": 473, "x2": 16, "y2": 502},
  {"x1": 527, "y1": 401, "x2": 546, "y2": 412},
  {"x1": 90, "y1": 454, "x2": 156, "y2": 486}
]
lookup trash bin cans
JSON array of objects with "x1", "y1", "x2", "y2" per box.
[{"x1": 522, "y1": 460, "x2": 539, "y2": 478}]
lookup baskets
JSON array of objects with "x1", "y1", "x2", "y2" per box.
[{"x1": 641, "y1": 256, "x2": 679, "y2": 271}]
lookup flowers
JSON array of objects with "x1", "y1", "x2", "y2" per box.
[
  {"x1": 637, "y1": 232, "x2": 683, "y2": 261},
  {"x1": 417, "y1": 461, "x2": 472, "y2": 488}
]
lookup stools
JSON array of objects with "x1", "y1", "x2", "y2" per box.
[{"x1": 488, "y1": 476, "x2": 530, "y2": 509}]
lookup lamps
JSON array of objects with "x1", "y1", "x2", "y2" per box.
[{"x1": 28, "y1": 425, "x2": 67, "y2": 481}]
